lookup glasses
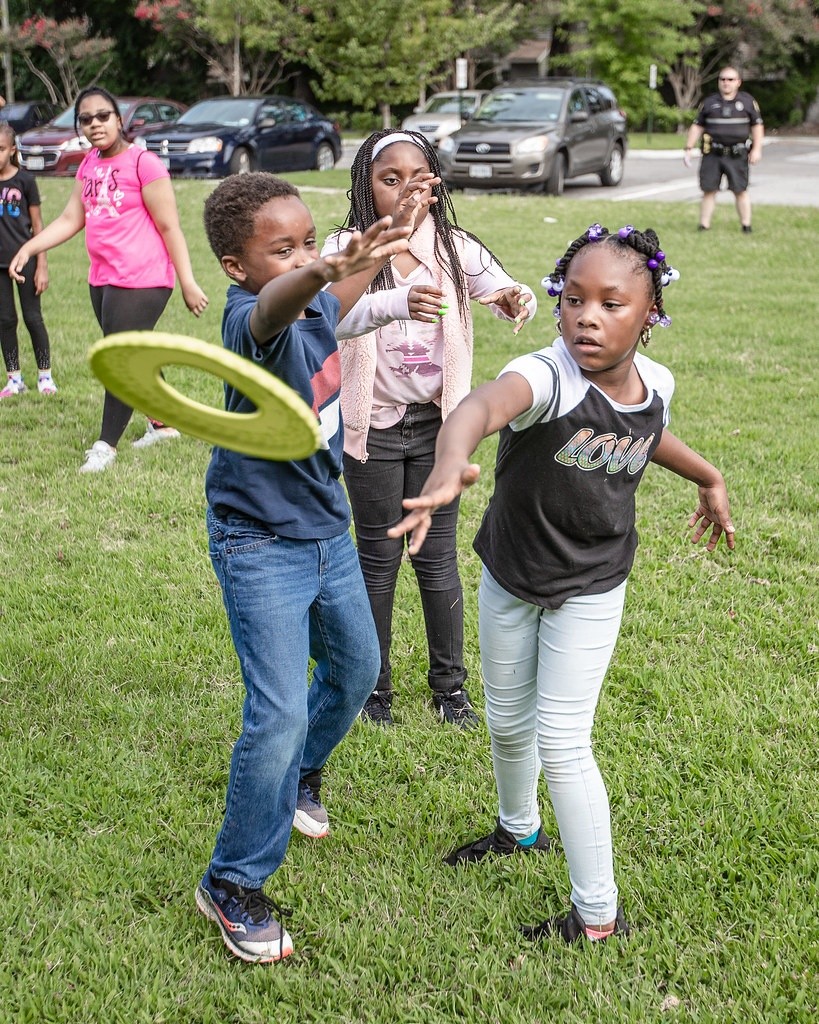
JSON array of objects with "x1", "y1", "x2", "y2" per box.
[
  {"x1": 718, "y1": 77, "x2": 739, "y2": 81},
  {"x1": 79, "y1": 111, "x2": 116, "y2": 125}
]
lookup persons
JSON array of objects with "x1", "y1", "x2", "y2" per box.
[
  {"x1": 320, "y1": 129, "x2": 538, "y2": 730},
  {"x1": 0, "y1": 124, "x2": 58, "y2": 398},
  {"x1": 683, "y1": 65, "x2": 764, "y2": 234},
  {"x1": 9, "y1": 87, "x2": 209, "y2": 475},
  {"x1": 388, "y1": 223, "x2": 736, "y2": 950},
  {"x1": 193, "y1": 172, "x2": 442, "y2": 963}
]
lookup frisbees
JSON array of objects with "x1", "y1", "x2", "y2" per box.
[{"x1": 86, "y1": 331, "x2": 322, "y2": 462}]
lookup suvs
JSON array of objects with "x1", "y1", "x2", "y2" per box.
[{"x1": 434, "y1": 76, "x2": 630, "y2": 197}]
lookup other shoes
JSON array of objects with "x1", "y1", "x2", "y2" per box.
[
  {"x1": 0, "y1": 379, "x2": 28, "y2": 398},
  {"x1": 38, "y1": 377, "x2": 57, "y2": 396}
]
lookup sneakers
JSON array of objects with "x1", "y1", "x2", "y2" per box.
[
  {"x1": 80, "y1": 440, "x2": 118, "y2": 474},
  {"x1": 434, "y1": 683, "x2": 481, "y2": 732},
  {"x1": 195, "y1": 869, "x2": 294, "y2": 966},
  {"x1": 360, "y1": 690, "x2": 393, "y2": 726},
  {"x1": 293, "y1": 770, "x2": 329, "y2": 839},
  {"x1": 446, "y1": 815, "x2": 550, "y2": 866},
  {"x1": 515, "y1": 903, "x2": 632, "y2": 947},
  {"x1": 133, "y1": 422, "x2": 182, "y2": 448}
]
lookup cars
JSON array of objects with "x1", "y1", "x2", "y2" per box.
[
  {"x1": 0, "y1": 100, "x2": 66, "y2": 137},
  {"x1": 401, "y1": 90, "x2": 492, "y2": 149},
  {"x1": 132, "y1": 92, "x2": 342, "y2": 180},
  {"x1": 13, "y1": 97, "x2": 189, "y2": 178}
]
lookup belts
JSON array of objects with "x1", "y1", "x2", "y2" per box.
[{"x1": 703, "y1": 147, "x2": 735, "y2": 156}]
